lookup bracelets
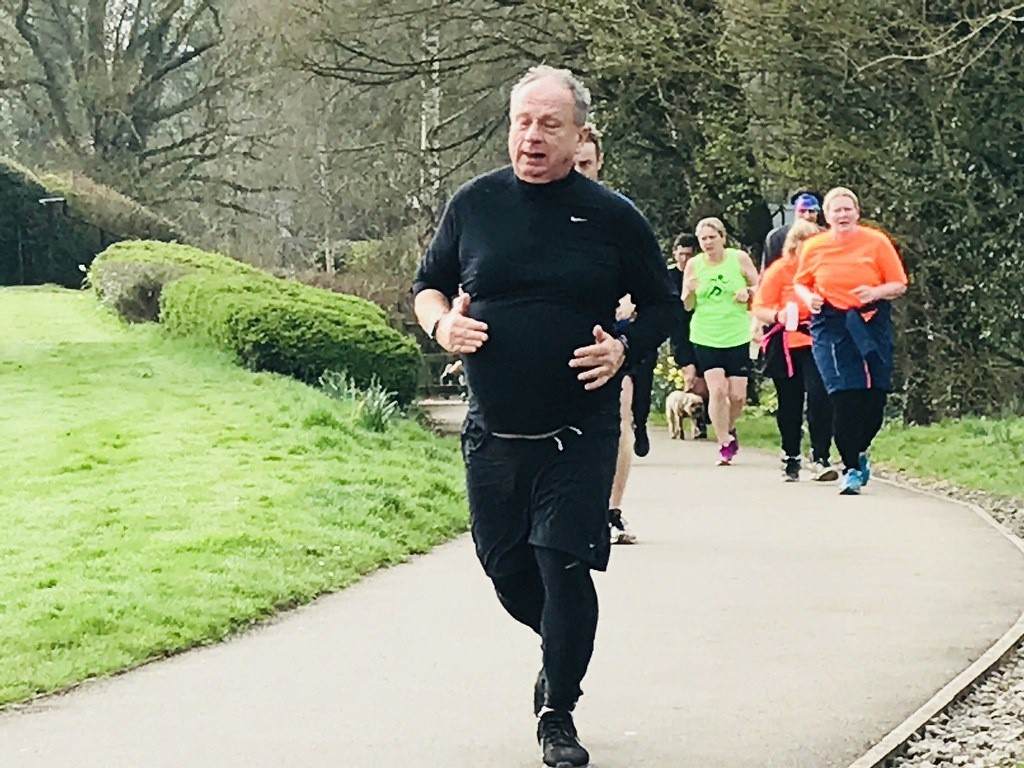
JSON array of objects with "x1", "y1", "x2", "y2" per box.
[
  {"x1": 431, "y1": 319, "x2": 439, "y2": 341},
  {"x1": 618, "y1": 334, "x2": 629, "y2": 350}
]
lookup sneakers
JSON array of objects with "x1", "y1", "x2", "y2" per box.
[{"x1": 537, "y1": 710, "x2": 590, "y2": 768}]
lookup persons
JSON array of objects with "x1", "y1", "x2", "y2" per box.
[
  {"x1": 666, "y1": 231, "x2": 714, "y2": 440},
  {"x1": 629, "y1": 352, "x2": 661, "y2": 458},
  {"x1": 750, "y1": 221, "x2": 840, "y2": 480},
  {"x1": 573, "y1": 122, "x2": 637, "y2": 543},
  {"x1": 761, "y1": 194, "x2": 826, "y2": 273},
  {"x1": 412, "y1": 66, "x2": 685, "y2": 768},
  {"x1": 793, "y1": 185, "x2": 908, "y2": 495},
  {"x1": 681, "y1": 216, "x2": 759, "y2": 465}
]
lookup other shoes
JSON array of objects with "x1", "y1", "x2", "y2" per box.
[
  {"x1": 839, "y1": 469, "x2": 863, "y2": 495},
  {"x1": 716, "y1": 443, "x2": 732, "y2": 466},
  {"x1": 728, "y1": 428, "x2": 738, "y2": 451},
  {"x1": 610, "y1": 508, "x2": 636, "y2": 544},
  {"x1": 781, "y1": 454, "x2": 802, "y2": 482},
  {"x1": 860, "y1": 452, "x2": 870, "y2": 486},
  {"x1": 633, "y1": 426, "x2": 650, "y2": 457},
  {"x1": 810, "y1": 457, "x2": 839, "y2": 481}
]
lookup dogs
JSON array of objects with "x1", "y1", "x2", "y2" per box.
[{"x1": 665, "y1": 391, "x2": 705, "y2": 439}]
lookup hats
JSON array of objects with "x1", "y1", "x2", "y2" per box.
[
  {"x1": 795, "y1": 194, "x2": 820, "y2": 211},
  {"x1": 674, "y1": 232, "x2": 697, "y2": 255}
]
